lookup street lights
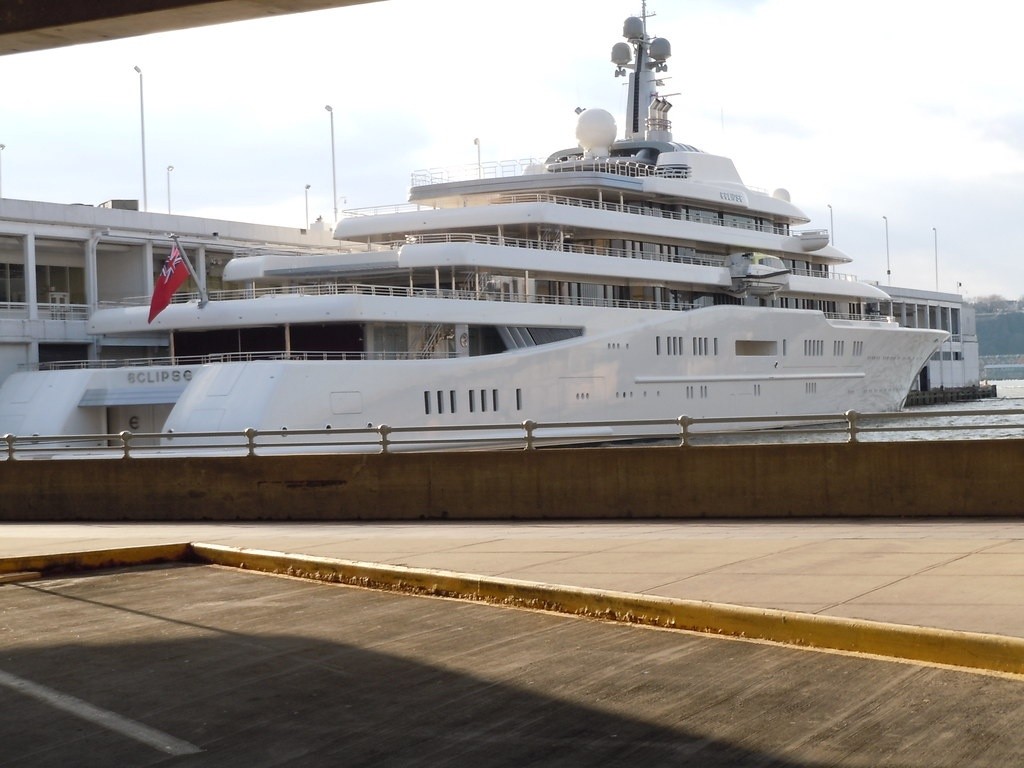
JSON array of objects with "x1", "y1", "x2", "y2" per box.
[
  {"x1": 882, "y1": 215, "x2": 890, "y2": 285},
  {"x1": 166, "y1": 165, "x2": 174, "y2": 215},
  {"x1": 132, "y1": 65, "x2": 147, "y2": 213},
  {"x1": 932, "y1": 227, "x2": 939, "y2": 291},
  {"x1": 827, "y1": 204, "x2": 834, "y2": 247},
  {"x1": 325, "y1": 105, "x2": 337, "y2": 223},
  {"x1": 474, "y1": 138, "x2": 481, "y2": 179},
  {"x1": 305, "y1": 183, "x2": 311, "y2": 230}
]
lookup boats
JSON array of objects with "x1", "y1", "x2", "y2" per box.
[{"x1": 0, "y1": 0, "x2": 955, "y2": 452}]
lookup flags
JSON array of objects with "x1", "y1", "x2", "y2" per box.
[{"x1": 148, "y1": 245, "x2": 191, "y2": 324}]
